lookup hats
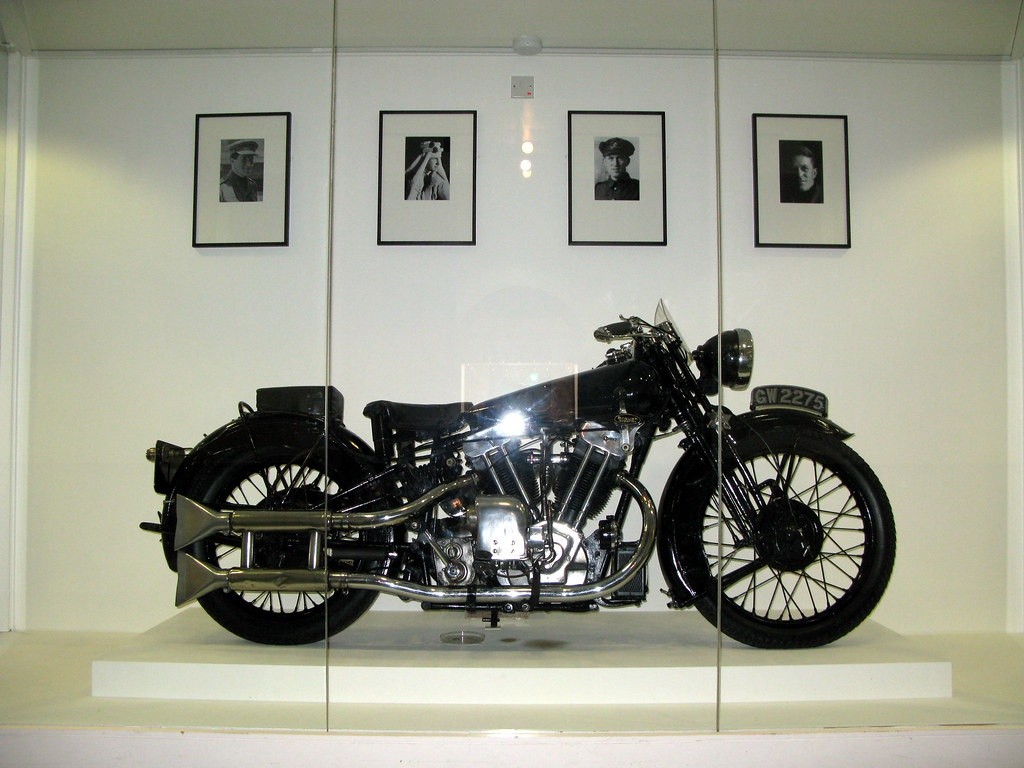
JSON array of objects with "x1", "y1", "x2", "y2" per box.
[
  {"x1": 420, "y1": 141, "x2": 443, "y2": 158},
  {"x1": 599, "y1": 138, "x2": 635, "y2": 158},
  {"x1": 226, "y1": 140, "x2": 258, "y2": 155}
]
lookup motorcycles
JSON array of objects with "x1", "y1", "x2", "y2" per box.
[{"x1": 140, "y1": 298, "x2": 899, "y2": 651}]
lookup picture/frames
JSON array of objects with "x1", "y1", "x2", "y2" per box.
[
  {"x1": 567, "y1": 110, "x2": 667, "y2": 246},
  {"x1": 377, "y1": 110, "x2": 478, "y2": 246},
  {"x1": 751, "y1": 113, "x2": 851, "y2": 249},
  {"x1": 191, "y1": 112, "x2": 289, "y2": 246}
]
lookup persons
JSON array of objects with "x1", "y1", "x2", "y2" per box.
[
  {"x1": 405, "y1": 141, "x2": 450, "y2": 200},
  {"x1": 219, "y1": 140, "x2": 259, "y2": 202},
  {"x1": 780, "y1": 146, "x2": 824, "y2": 203},
  {"x1": 595, "y1": 138, "x2": 639, "y2": 200}
]
